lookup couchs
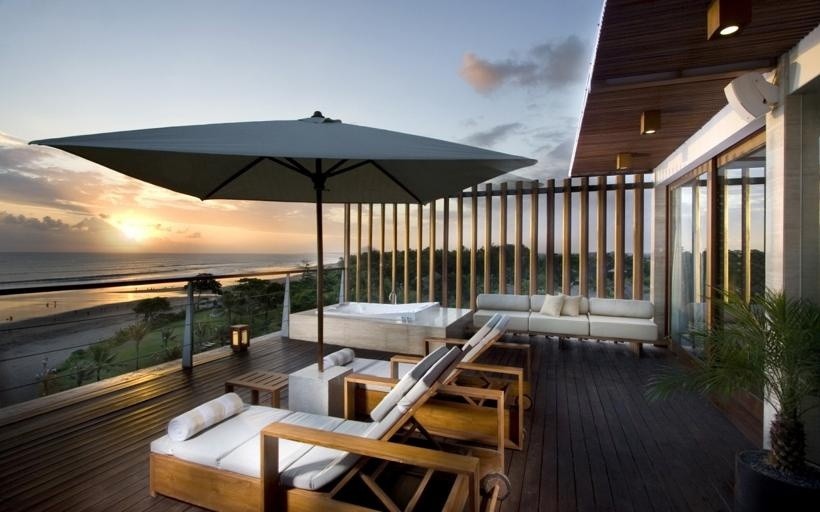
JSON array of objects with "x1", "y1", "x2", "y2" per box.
[{"x1": 473, "y1": 293, "x2": 658, "y2": 360}]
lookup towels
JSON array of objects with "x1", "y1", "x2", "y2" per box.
[
  {"x1": 322, "y1": 348, "x2": 355, "y2": 366},
  {"x1": 168, "y1": 392, "x2": 243, "y2": 442}
]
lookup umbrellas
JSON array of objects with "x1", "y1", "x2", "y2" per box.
[{"x1": 28, "y1": 111, "x2": 537, "y2": 371}]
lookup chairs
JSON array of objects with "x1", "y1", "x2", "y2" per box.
[
  {"x1": 149, "y1": 344, "x2": 511, "y2": 511},
  {"x1": 288, "y1": 314, "x2": 532, "y2": 451}
]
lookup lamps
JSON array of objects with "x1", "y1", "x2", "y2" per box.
[
  {"x1": 707, "y1": 0, "x2": 746, "y2": 40},
  {"x1": 640, "y1": 110, "x2": 661, "y2": 135},
  {"x1": 617, "y1": 153, "x2": 632, "y2": 170},
  {"x1": 230, "y1": 324, "x2": 250, "y2": 353}
]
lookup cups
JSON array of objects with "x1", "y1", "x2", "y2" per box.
[
  {"x1": 407, "y1": 317, "x2": 413, "y2": 323},
  {"x1": 402, "y1": 317, "x2": 406, "y2": 323}
]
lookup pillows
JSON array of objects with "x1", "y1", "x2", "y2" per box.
[
  {"x1": 539, "y1": 294, "x2": 565, "y2": 317},
  {"x1": 558, "y1": 293, "x2": 583, "y2": 317}
]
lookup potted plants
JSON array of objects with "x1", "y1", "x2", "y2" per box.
[{"x1": 642, "y1": 283, "x2": 820, "y2": 512}]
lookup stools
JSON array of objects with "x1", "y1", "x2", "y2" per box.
[{"x1": 226, "y1": 370, "x2": 288, "y2": 408}]
